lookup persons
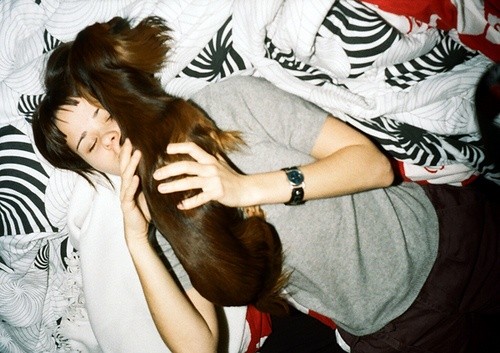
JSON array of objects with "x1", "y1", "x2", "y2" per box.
[{"x1": 31, "y1": 72, "x2": 500, "y2": 353}]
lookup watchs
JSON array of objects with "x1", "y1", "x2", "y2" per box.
[{"x1": 281, "y1": 165, "x2": 305, "y2": 208}]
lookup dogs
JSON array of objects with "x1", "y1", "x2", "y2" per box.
[{"x1": 66, "y1": 15, "x2": 299, "y2": 317}]
